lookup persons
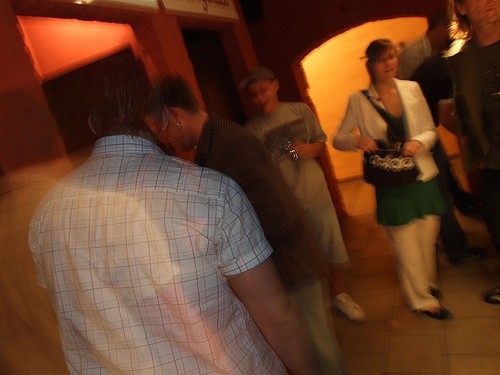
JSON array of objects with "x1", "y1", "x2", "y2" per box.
[
  {"x1": 397, "y1": 0, "x2": 500, "y2": 305},
  {"x1": 146, "y1": 70, "x2": 344, "y2": 375},
  {"x1": 239, "y1": 65, "x2": 364, "y2": 323},
  {"x1": 332, "y1": 39, "x2": 455, "y2": 320},
  {"x1": 27, "y1": 57, "x2": 318, "y2": 375}
]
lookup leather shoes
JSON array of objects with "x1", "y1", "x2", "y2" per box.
[
  {"x1": 430, "y1": 286, "x2": 444, "y2": 300},
  {"x1": 412, "y1": 304, "x2": 453, "y2": 319}
]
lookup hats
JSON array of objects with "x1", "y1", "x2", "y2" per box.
[{"x1": 240, "y1": 66, "x2": 275, "y2": 89}]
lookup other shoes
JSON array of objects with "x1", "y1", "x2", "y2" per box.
[{"x1": 484, "y1": 287, "x2": 500, "y2": 304}]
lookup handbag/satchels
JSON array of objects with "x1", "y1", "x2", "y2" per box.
[{"x1": 363, "y1": 147, "x2": 417, "y2": 186}]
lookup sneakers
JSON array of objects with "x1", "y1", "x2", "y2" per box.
[{"x1": 331, "y1": 293, "x2": 365, "y2": 321}]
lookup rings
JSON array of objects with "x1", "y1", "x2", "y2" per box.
[{"x1": 289, "y1": 148, "x2": 300, "y2": 161}]
